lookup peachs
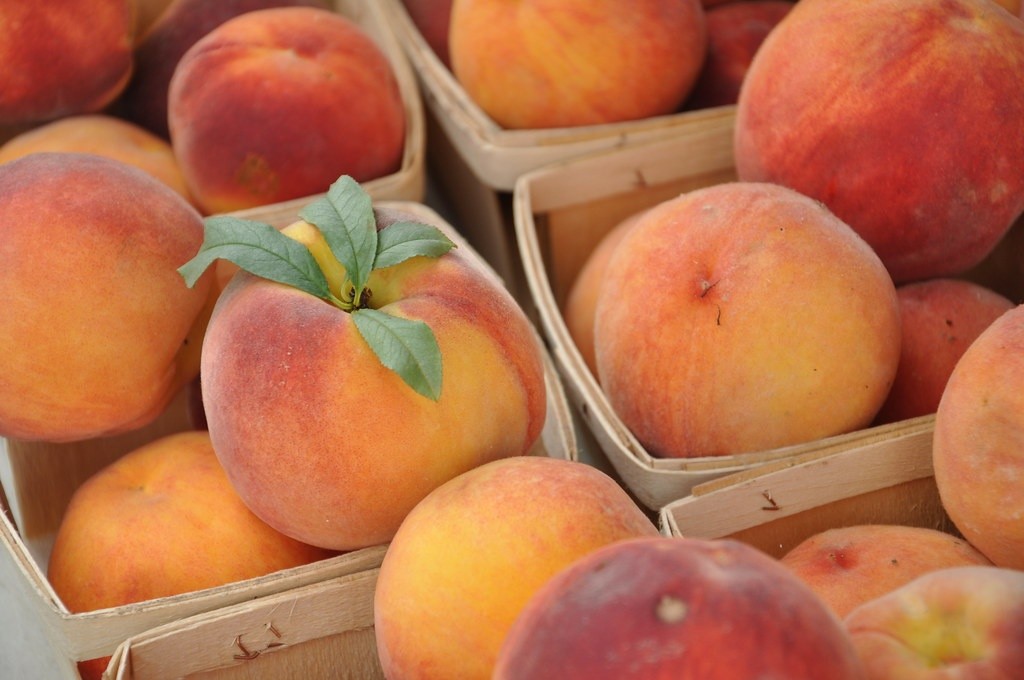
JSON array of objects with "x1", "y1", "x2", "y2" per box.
[{"x1": 0, "y1": 0, "x2": 1024, "y2": 680}]
